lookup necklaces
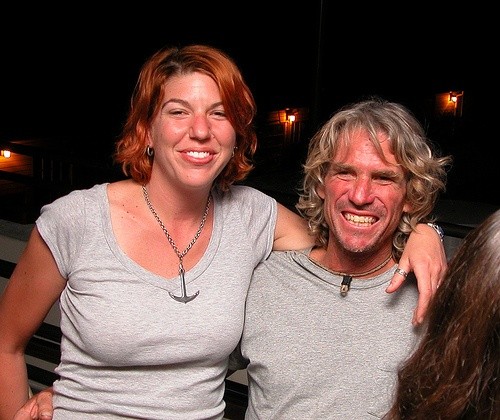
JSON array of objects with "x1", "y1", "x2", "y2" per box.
[
  {"x1": 143, "y1": 184, "x2": 211, "y2": 304},
  {"x1": 305, "y1": 253, "x2": 393, "y2": 297}
]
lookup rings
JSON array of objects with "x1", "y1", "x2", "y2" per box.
[{"x1": 396, "y1": 268, "x2": 407, "y2": 277}]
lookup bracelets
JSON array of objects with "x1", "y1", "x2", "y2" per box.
[{"x1": 428, "y1": 223, "x2": 445, "y2": 242}]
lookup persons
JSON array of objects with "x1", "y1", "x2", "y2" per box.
[
  {"x1": 0, "y1": 46, "x2": 448, "y2": 420},
  {"x1": 225, "y1": 101, "x2": 452, "y2": 420},
  {"x1": 382, "y1": 210, "x2": 500, "y2": 420}
]
